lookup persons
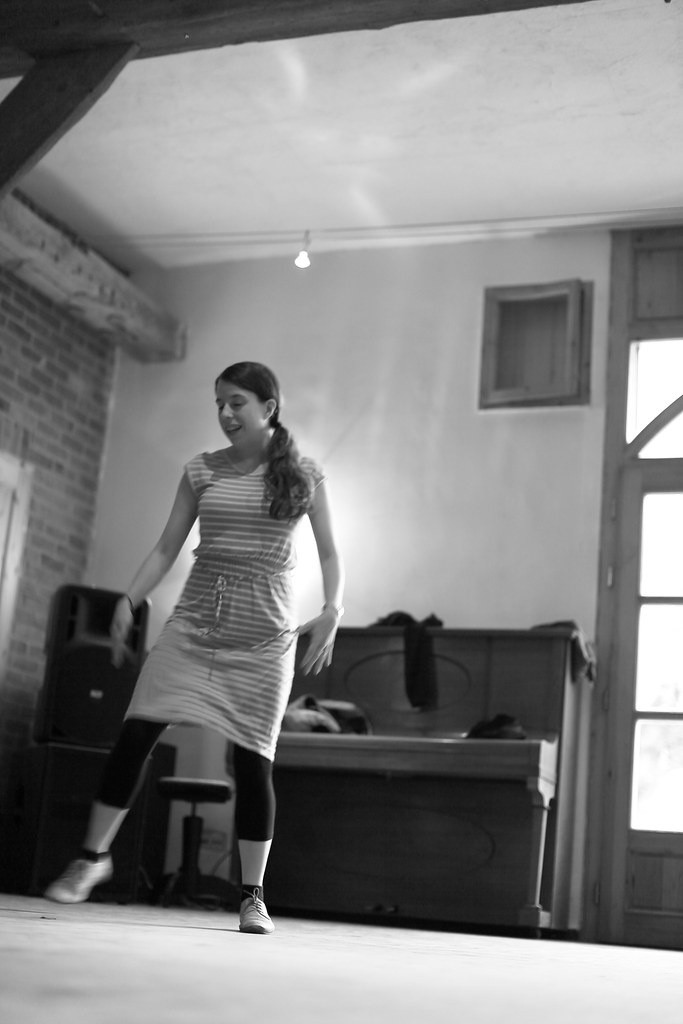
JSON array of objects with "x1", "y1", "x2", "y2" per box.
[{"x1": 46, "y1": 362, "x2": 343, "y2": 933}]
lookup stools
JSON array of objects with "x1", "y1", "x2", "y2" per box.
[{"x1": 153, "y1": 777, "x2": 232, "y2": 911}]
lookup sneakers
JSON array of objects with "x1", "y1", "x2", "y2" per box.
[
  {"x1": 239, "y1": 888, "x2": 275, "y2": 934},
  {"x1": 45, "y1": 858, "x2": 113, "y2": 903}
]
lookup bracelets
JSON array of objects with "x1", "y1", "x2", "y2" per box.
[
  {"x1": 323, "y1": 602, "x2": 344, "y2": 616},
  {"x1": 126, "y1": 594, "x2": 134, "y2": 612}
]
